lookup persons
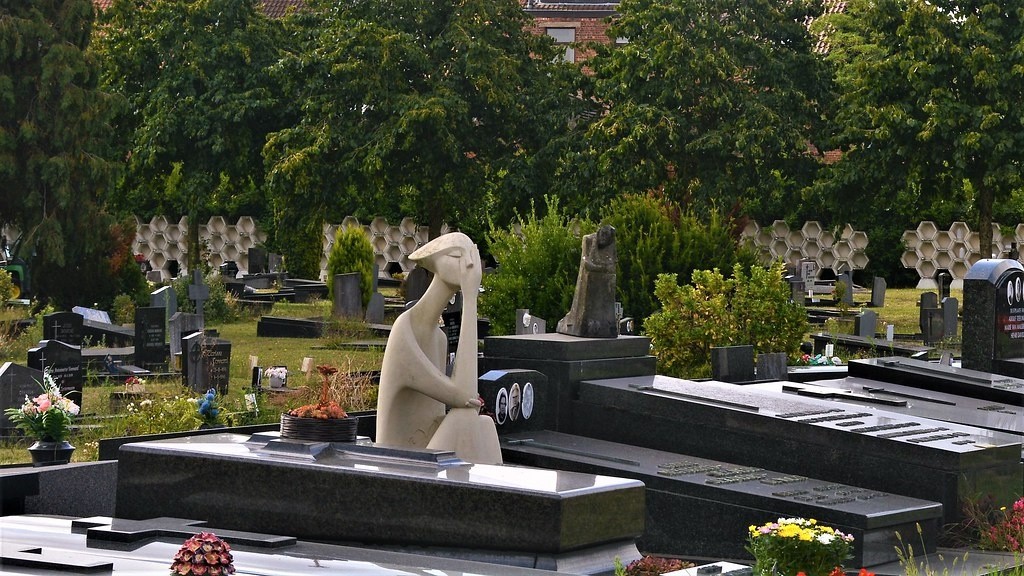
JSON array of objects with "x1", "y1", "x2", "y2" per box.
[{"x1": 375, "y1": 230, "x2": 505, "y2": 466}]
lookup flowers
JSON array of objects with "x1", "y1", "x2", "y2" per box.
[
  {"x1": 286, "y1": 356, "x2": 350, "y2": 420},
  {"x1": 3, "y1": 361, "x2": 82, "y2": 444},
  {"x1": 125, "y1": 375, "x2": 146, "y2": 384},
  {"x1": 195, "y1": 386, "x2": 221, "y2": 424},
  {"x1": 263, "y1": 366, "x2": 287, "y2": 380}
]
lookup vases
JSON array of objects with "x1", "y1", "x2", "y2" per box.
[
  {"x1": 268, "y1": 375, "x2": 283, "y2": 389},
  {"x1": 197, "y1": 422, "x2": 226, "y2": 429},
  {"x1": 27, "y1": 440, "x2": 76, "y2": 464},
  {"x1": 125, "y1": 383, "x2": 145, "y2": 393},
  {"x1": 278, "y1": 411, "x2": 359, "y2": 442}
]
